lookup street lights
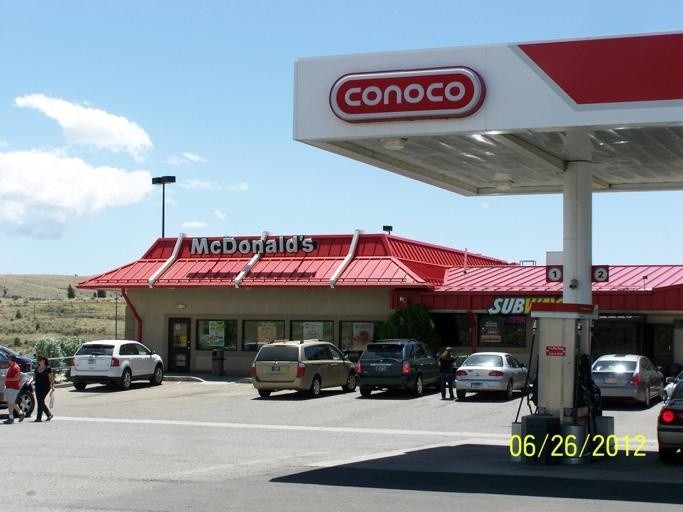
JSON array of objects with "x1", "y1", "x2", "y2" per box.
[{"x1": 151, "y1": 174, "x2": 175, "y2": 238}]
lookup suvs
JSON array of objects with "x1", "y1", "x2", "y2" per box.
[{"x1": 0, "y1": 351, "x2": 37, "y2": 417}]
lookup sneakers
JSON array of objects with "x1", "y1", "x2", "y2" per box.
[
  {"x1": 45, "y1": 414, "x2": 53, "y2": 421},
  {"x1": 3, "y1": 419, "x2": 13, "y2": 423},
  {"x1": 18, "y1": 411, "x2": 26, "y2": 422}
]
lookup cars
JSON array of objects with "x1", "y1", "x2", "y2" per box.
[
  {"x1": 251, "y1": 339, "x2": 357, "y2": 397},
  {"x1": 69, "y1": 339, "x2": 164, "y2": 390},
  {"x1": 661, "y1": 369, "x2": 682, "y2": 402},
  {"x1": 657, "y1": 382, "x2": 682, "y2": 459},
  {"x1": 590, "y1": 353, "x2": 666, "y2": 406},
  {"x1": 0, "y1": 345, "x2": 34, "y2": 375},
  {"x1": 454, "y1": 352, "x2": 530, "y2": 400}
]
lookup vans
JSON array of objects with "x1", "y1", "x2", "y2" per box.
[{"x1": 354, "y1": 338, "x2": 443, "y2": 397}]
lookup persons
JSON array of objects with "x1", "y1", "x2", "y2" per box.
[
  {"x1": 26, "y1": 354, "x2": 54, "y2": 424},
  {"x1": 438, "y1": 347, "x2": 458, "y2": 400},
  {"x1": 3, "y1": 354, "x2": 26, "y2": 425}
]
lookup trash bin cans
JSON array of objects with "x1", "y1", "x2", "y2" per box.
[{"x1": 212, "y1": 348, "x2": 224, "y2": 376}]
